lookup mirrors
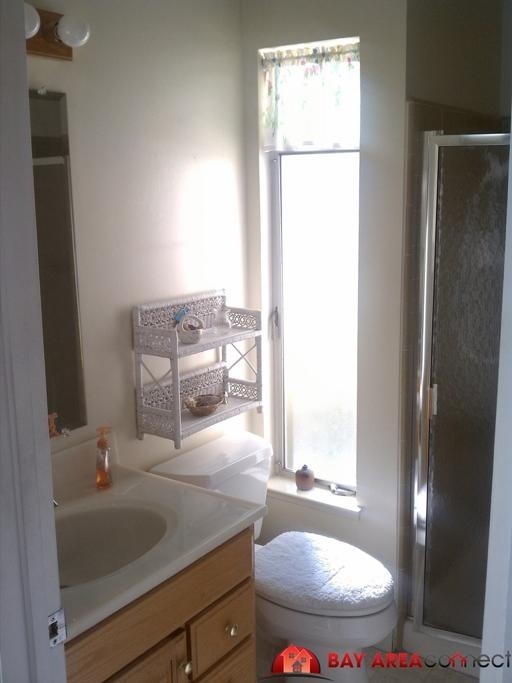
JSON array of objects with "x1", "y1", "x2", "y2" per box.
[{"x1": 30, "y1": 89, "x2": 89, "y2": 442}]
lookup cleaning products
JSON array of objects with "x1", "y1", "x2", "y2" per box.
[
  {"x1": 93, "y1": 425, "x2": 113, "y2": 491},
  {"x1": 46, "y1": 410, "x2": 59, "y2": 438}
]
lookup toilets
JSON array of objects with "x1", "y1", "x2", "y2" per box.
[{"x1": 146, "y1": 430, "x2": 398, "y2": 682}]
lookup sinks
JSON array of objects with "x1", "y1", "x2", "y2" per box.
[{"x1": 55, "y1": 503, "x2": 167, "y2": 589}]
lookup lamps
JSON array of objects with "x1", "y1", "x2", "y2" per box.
[{"x1": 23, "y1": 2, "x2": 90, "y2": 60}]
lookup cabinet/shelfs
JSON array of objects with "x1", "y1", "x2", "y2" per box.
[
  {"x1": 64, "y1": 524, "x2": 263, "y2": 683},
  {"x1": 130, "y1": 287, "x2": 264, "y2": 449}
]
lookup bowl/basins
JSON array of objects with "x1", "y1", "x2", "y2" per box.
[{"x1": 185, "y1": 393, "x2": 222, "y2": 417}]
